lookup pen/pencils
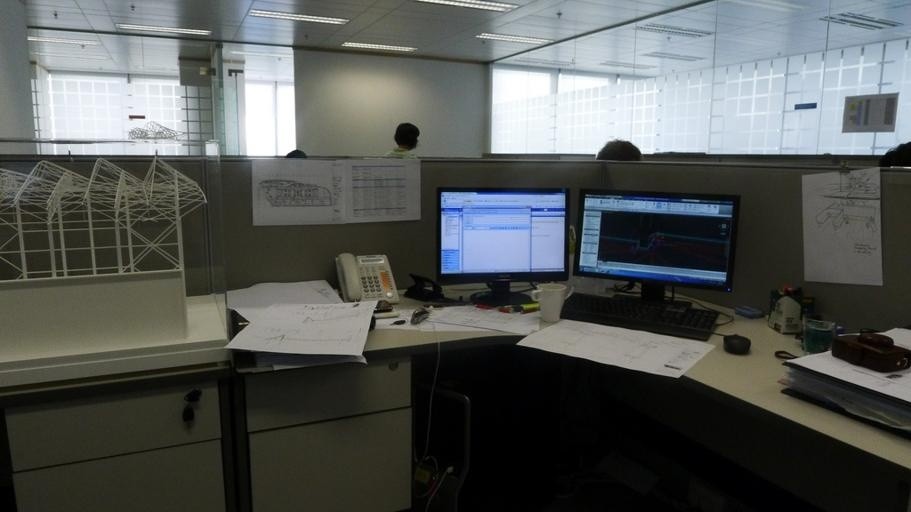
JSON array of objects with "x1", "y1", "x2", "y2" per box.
[{"x1": 475, "y1": 302, "x2": 540, "y2": 314}]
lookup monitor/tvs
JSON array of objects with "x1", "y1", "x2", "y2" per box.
[
  {"x1": 437, "y1": 186, "x2": 571, "y2": 307},
  {"x1": 573, "y1": 188, "x2": 741, "y2": 308}
]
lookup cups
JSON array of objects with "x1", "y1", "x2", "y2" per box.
[
  {"x1": 533, "y1": 283, "x2": 566, "y2": 324},
  {"x1": 803, "y1": 314, "x2": 835, "y2": 355}
]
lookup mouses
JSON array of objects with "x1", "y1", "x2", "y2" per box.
[{"x1": 723, "y1": 334, "x2": 751, "y2": 354}]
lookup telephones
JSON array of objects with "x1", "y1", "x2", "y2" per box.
[{"x1": 335, "y1": 253, "x2": 400, "y2": 304}]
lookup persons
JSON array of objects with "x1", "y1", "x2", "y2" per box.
[
  {"x1": 595, "y1": 139, "x2": 642, "y2": 161},
  {"x1": 384, "y1": 122, "x2": 421, "y2": 159},
  {"x1": 879, "y1": 140, "x2": 911, "y2": 167},
  {"x1": 286, "y1": 149, "x2": 307, "y2": 158}
]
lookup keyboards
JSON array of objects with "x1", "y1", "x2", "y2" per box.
[{"x1": 561, "y1": 292, "x2": 720, "y2": 341}]
